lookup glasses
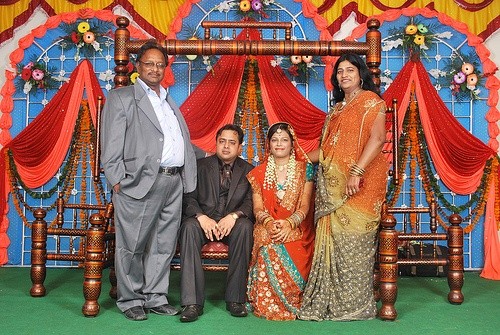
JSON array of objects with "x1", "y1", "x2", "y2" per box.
[{"x1": 138, "y1": 60, "x2": 167, "y2": 69}]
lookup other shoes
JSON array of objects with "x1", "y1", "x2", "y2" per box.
[
  {"x1": 144, "y1": 304, "x2": 181, "y2": 316},
  {"x1": 124, "y1": 305, "x2": 147, "y2": 321},
  {"x1": 225, "y1": 302, "x2": 249, "y2": 317},
  {"x1": 181, "y1": 304, "x2": 203, "y2": 322}
]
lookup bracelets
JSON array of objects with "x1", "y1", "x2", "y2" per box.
[
  {"x1": 255, "y1": 210, "x2": 275, "y2": 229},
  {"x1": 348, "y1": 163, "x2": 366, "y2": 178},
  {"x1": 285, "y1": 209, "x2": 307, "y2": 230}
]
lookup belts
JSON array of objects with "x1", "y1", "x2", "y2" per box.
[{"x1": 158, "y1": 166, "x2": 183, "y2": 175}]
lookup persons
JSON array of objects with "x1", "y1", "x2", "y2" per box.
[
  {"x1": 244, "y1": 121, "x2": 316, "y2": 322},
  {"x1": 100, "y1": 37, "x2": 216, "y2": 322},
  {"x1": 178, "y1": 123, "x2": 257, "y2": 323},
  {"x1": 295, "y1": 50, "x2": 389, "y2": 325}
]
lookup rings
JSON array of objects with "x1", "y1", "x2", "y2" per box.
[
  {"x1": 205, "y1": 223, "x2": 219, "y2": 234},
  {"x1": 348, "y1": 188, "x2": 356, "y2": 193},
  {"x1": 277, "y1": 228, "x2": 281, "y2": 236}
]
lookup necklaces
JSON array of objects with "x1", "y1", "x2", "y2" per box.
[
  {"x1": 338, "y1": 89, "x2": 362, "y2": 110},
  {"x1": 275, "y1": 159, "x2": 289, "y2": 172}
]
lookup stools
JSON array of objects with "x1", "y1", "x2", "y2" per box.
[{"x1": 171, "y1": 243, "x2": 228, "y2": 270}]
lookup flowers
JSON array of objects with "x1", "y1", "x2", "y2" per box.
[
  {"x1": 444, "y1": 56, "x2": 482, "y2": 102},
  {"x1": 216, "y1": 0, "x2": 275, "y2": 19},
  {"x1": 14, "y1": 54, "x2": 54, "y2": 96},
  {"x1": 387, "y1": 17, "x2": 437, "y2": 59},
  {"x1": 56, "y1": 18, "x2": 109, "y2": 52},
  {"x1": 271, "y1": 55, "x2": 321, "y2": 84},
  {"x1": 127, "y1": 58, "x2": 140, "y2": 83}
]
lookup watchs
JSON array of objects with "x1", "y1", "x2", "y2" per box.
[{"x1": 229, "y1": 211, "x2": 240, "y2": 220}]
